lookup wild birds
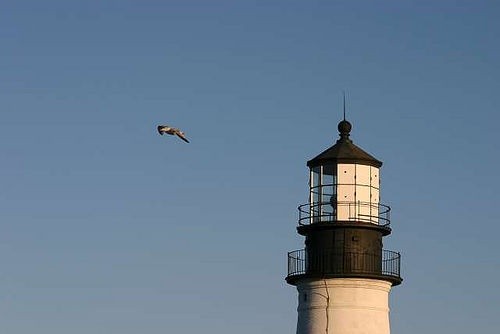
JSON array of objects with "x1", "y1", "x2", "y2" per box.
[{"x1": 157, "y1": 125, "x2": 189, "y2": 145}]
